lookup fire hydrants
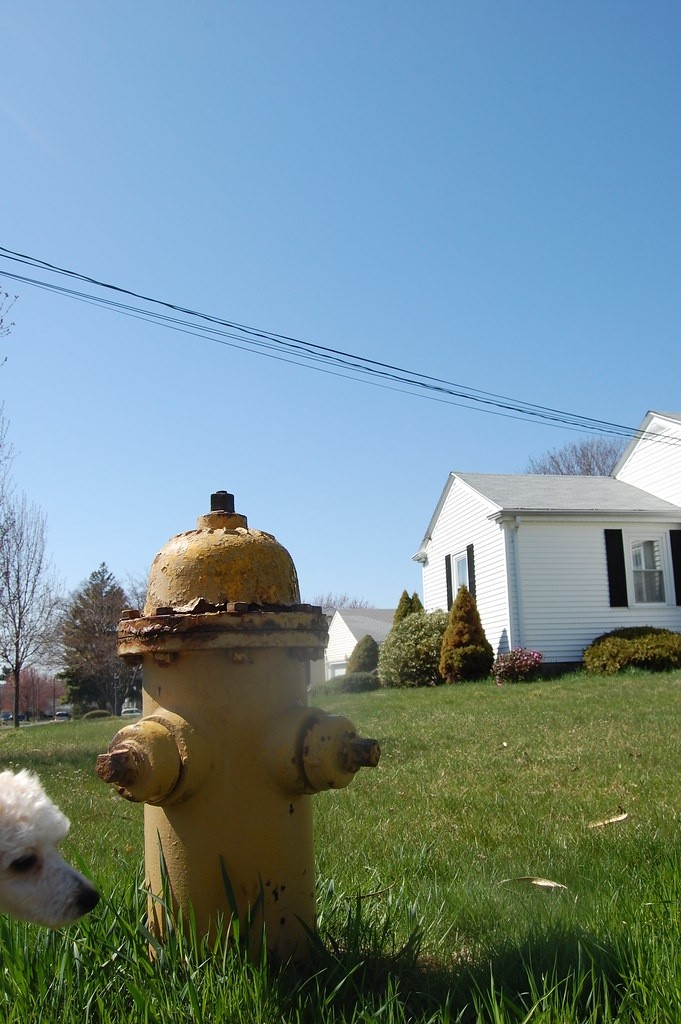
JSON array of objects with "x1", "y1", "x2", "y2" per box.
[{"x1": 93, "y1": 490, "x2": 380, "y2": 982}]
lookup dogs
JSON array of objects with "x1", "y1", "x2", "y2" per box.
[{"x1": 0, "y1": 766, "x2": 102, "y2": 927}]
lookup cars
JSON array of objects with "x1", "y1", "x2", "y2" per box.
[
  {"x1": 6, "y1": 714, "x2": 28, "y2": 721},
  {"x1": 54, "y1": 711, "x2": 71, "y2": 722},
  {"x1": 121, "y1": 708, "x2": 142, "y2": 716}
]
